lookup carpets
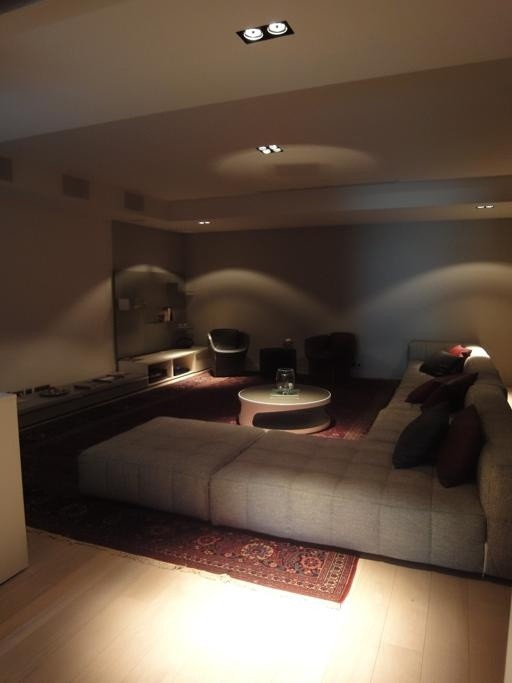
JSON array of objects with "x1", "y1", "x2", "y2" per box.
[{"x1": 20, "y1": 369, "x2": 401, "y2": 603}]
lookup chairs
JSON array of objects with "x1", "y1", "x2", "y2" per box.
[
  {"x1": 207, "y1": 328, "x2": 249, "y2": 376},
  {"x1": 367, "y1": 385, "x2": 512, "y2": 443},
  {"x1": 305, "y1": 332, "x2": 355, "y2": 377}
]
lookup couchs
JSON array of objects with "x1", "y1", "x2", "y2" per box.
[{"x1": 366, "y1": 340, "x2": 507, "y2": 408}]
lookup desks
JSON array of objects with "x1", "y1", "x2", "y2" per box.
[{"x1": 259, "y1": 348, "x2": 296, "y2": 378}]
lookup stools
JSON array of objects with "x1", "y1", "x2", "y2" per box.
[{"x1": 78, "y1": 415, "x2": 266, "y2": 522}]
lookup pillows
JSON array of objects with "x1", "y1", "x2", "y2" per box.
[{"x1": 392, "y1": 344, "x2": 481, "y2": 488}]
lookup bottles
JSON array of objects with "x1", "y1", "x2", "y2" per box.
[{"x1": 275, "y1": 367, "x2": 296, "y2": 395}]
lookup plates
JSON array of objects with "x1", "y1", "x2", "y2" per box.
[{"x1": 39, "y1": 388, "x2": 71, "y2": 396}]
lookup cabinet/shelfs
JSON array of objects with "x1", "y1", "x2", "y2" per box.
[
  {"x1": 118, "y1": 345, "x2": 209, "y2": 388},
  {"x1": 17, "y1": 372, "x2": 148, "y2": 430}
]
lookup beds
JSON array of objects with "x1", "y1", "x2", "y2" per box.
[{"x1": 210, "y1": 428, "x2": 512, "y2": 581}]
